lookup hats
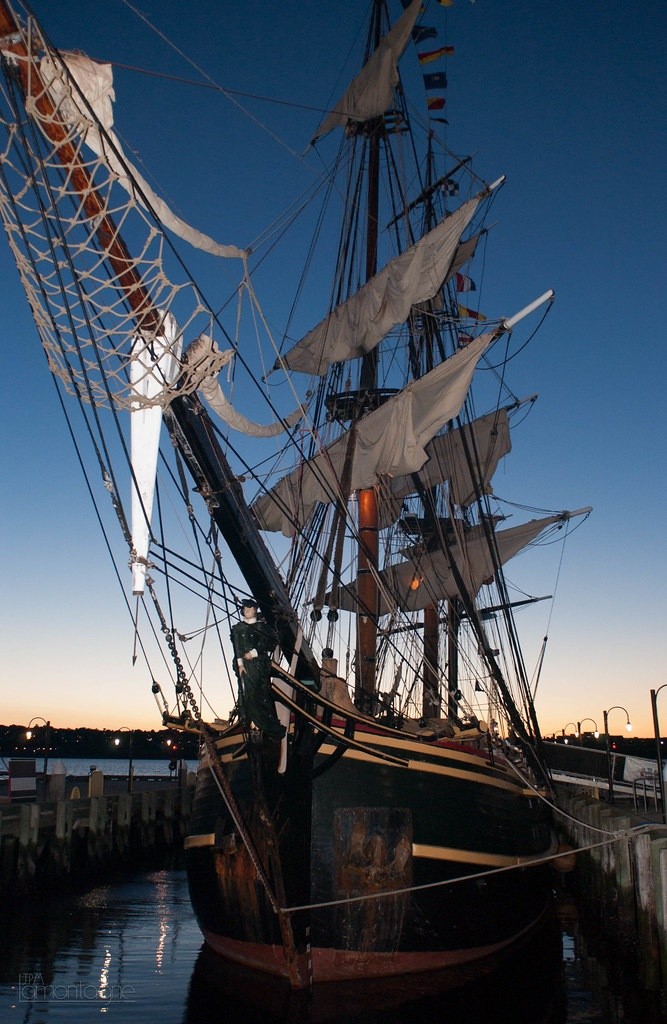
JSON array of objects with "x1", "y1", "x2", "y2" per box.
[{"x1": 240, "y1": 599, "x2": 259, "y2": 615}]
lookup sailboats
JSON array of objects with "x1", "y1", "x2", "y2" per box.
[{"x1": 1, "y1": 0, "x2": 597, "y2": 1013}]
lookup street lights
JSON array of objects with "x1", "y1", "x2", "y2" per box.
[
  {"x1": 26, "y1": 717, "x2": 50, "y2": 801},
  {"x1": 561, "y1": 722, "x2": 579, "y2": 745},
  {"x1": 112, "y1": 726, "x2": 133, "y2": 793},
  {"x1": 603, "y1": 706, "x2": 633, "y2": 806},
  {"x1": 578, "y1": 718, "x2": 599, "y2": 748},
  {"x1": 553, "y1": 729, "x2": 569, "y2": 745}
]
